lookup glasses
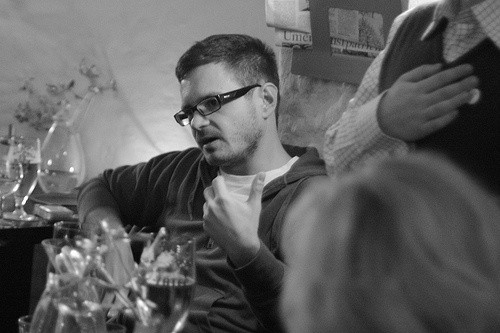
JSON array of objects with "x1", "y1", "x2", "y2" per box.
[{"x1": 174, "y1": 84, "x2": 262, "y2": 126}]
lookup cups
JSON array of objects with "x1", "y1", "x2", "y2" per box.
[
  {"x1": 18, "y1": 316, "x2": 33, "y2": 333},
  {"x1": 130, "y1": 232, "x2": 197, "y2": 333},
  {"x1": 47, "y1": 220, "x2": 108, "y2": 296}
]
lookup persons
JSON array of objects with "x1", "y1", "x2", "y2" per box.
[
  {"x1": 277, "y1": 152, "x2": 500, "y2": 333},
  {"x1": 324, "y1": 0, "x2": 500, "y2": 198},
  {"x1": 78, "y1": 33, "x2": 330, "y2": 333}
]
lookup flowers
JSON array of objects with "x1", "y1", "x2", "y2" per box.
[{"x1": 14, "y1": 58, "x2": 118, "y2": 133}]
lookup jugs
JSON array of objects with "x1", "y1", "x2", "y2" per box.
[
  {"x1": 37, "y1": 85, "x2": 100, "y2": 193},
  {"x1": 32, "y1": 238, "x2": 107, "y2": 333}
]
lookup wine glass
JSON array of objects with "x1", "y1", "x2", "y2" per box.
[
  {"x1": 0, "y1": 156, "x2": 24, "y2": 225},
  {"x1": 2, "y1": 135, "x2": 40, "y2": 221}
]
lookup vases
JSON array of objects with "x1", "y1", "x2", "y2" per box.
[{"x1": 37, "y1": 122, "x2": 87, "y2": 198}]
